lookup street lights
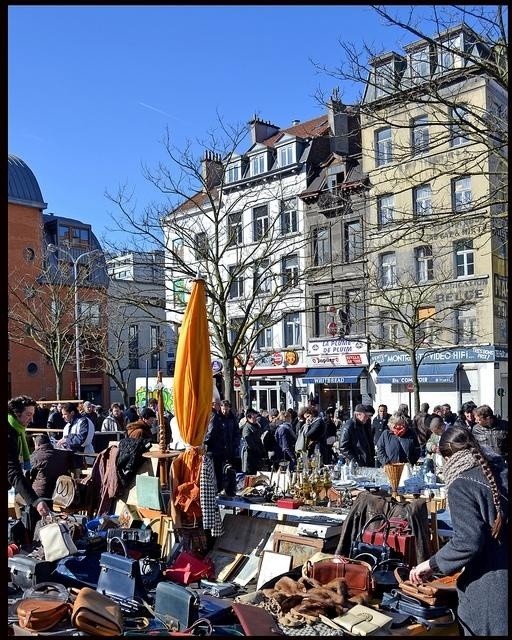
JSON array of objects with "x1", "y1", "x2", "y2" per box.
[{"x1": 47, "y1": 241, "x2": 100, "y2": 401}]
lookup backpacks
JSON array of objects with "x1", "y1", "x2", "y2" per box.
[{"x1": 116, "y1": 437, "x2": 151, "y2": 488}]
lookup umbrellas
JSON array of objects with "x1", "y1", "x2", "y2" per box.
[{"x1": 161, "y1": 266, "x2": 219, "y2": 562}]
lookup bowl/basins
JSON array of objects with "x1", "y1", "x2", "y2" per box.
[{"x1": 86, "y1": 515, "x2": 120, "y2": 536}]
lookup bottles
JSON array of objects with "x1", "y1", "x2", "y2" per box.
[{"x1": 325, "y1": 457, "x2": 436, "y2": 487}]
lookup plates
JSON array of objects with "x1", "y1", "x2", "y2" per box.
[{"x1": 240, "y1": 496, "x2": 271, "y2": 504}]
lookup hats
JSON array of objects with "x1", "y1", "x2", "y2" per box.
[
  {"x1": 268, "y1": 408, "x2": 280, "y2": 416},
  {"x1": 364, "y1": 405, "x2": 375, "y2": 414},
  {"x1": 355, "y1": 404, "x2": 370, "y2": 413},
  {"x1": 141, "y1": 408, "x2": 157, "y2": 418}
]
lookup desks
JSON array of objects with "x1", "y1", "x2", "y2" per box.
[{"x1": 214, "y1": 488, "x2": 359, "y2": 527}]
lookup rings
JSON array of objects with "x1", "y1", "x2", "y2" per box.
[{"x1": 411, "y1": 565, "x2": 416, "y2": 570}]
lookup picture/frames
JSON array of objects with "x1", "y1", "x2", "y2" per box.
[{"x1": 273, "y1": 530, "x2": 324, "y2": 568}]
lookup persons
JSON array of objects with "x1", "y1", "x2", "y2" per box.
[{"x1": 403, "y1": 423, "x2": 511, "y2": 635}]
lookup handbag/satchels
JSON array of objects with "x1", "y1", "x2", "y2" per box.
[
  {"x1": 380, "y1": 588, "x2": 456, "y2": 630},
  {"x1": 393, "y1": 567, "x2": 459, "y2": 606},
  {"x1": 233, "y1": 603, "x2": 283, "y2": 638},
  {"x1": 307, "y1": 558, "x2": 372, "y2": 597},
  {"x1": 139, "y1": 550, "x2": 239, "y2": 599},
  {"x1": 8, "y1": 504, "x2": 79, "y2": 563},
  {"x1": 8, "y1": 553, "x2": 52, "y2": 594},
  {"x1": 71, "y1": 587, "x2": 123, "y2": 637},
  {"x1": 347, "y1": 514, "x2": 416, "y2": 585},
  {"x1": 51, "y1": 474, "x2": 81, "y2": 509},
  {"x1": 154, "y1": 581, "x2": 201, "y2": 633},
  {"x1": 319, "y1": 604, "x2": 393, "y2": 637},
  {"x1": 16, "y1": 598, "x2": 68, "y2": 633},
  {"x1": 96, "y1": 536, "x2": 148, "y2": 603}
]
